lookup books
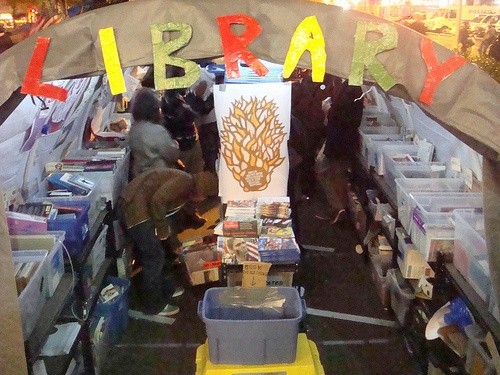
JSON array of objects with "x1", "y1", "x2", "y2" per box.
[
  {"x1": 3, "y1": 130, "x2": 127, "y2": 292},
  {"x1": 33, "y1": 225, "x2": 127, "y2": 375},
  {"x1": 375, "y1": 235, "x2": 393, "y2": 255},
  {"x1": 223, "y1": 198, "x2": 301, "y2": 270}
]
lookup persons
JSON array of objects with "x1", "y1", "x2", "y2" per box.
[
  {"x1": 288, "y1": 68, "x2": 366, "y2": 221},
  {"x1": 117, "y1": 168, "x2": 219, "y2": 303},
  {"x1": 130, "y1": 78, "x2": 220, "y2": 247}
]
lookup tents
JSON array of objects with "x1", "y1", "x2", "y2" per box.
[{"x1": 0, "y1": 0, "x2": 500, "y2": 375}]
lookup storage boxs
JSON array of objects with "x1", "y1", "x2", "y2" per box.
[
  {"x1": 8, "y1": 114, "x2": 325, "y2": 375},
  {"x1": 349, "y1": 125, "x2": 500, "y2": 375}
]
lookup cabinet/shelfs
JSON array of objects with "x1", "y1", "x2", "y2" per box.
[
  {"x1": 352, "y1": 155, "x2": 500, "y2": 373},
  {"x1": 24, "y1": 198, "x2": 119, "y2": 375}
]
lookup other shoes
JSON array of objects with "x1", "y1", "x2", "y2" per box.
[
  {"x1": 355, "y1": 210, "x2": 368, "y2": 239},
  {"x1": 158, "y1": 304, "x2": 179, "y2": 316},
  {"x1": 330, "y1": 207, "x2": 346, "y2": 224},
  {"x1": 172, "y1": 287, "x2": 185, "y2": 297}
]
